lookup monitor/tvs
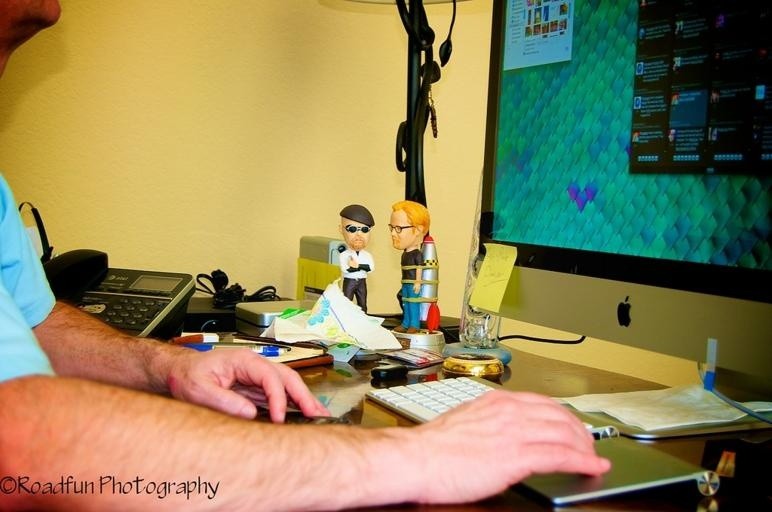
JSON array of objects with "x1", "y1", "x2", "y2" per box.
[{"x1": 479, "y1": 0, "x2": 772, "y2": 441}]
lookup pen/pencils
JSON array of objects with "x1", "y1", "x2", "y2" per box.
[{"x1": 183, "y1": 344, "x2": 282, "y2": 357}]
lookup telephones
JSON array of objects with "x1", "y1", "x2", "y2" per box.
[{"x1": 43, "y1": 249, "x2": 196, "y2": 337}]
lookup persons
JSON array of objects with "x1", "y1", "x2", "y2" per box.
[
  {"x1": 333, "y1": 202, "x2": 374, "y2": 317},
  {"x1": 0, "y1": 0, "x2": 611, "y2": 510},
  {"x1": 388, "y1": 199, "x2": 430, "y2": 338}
]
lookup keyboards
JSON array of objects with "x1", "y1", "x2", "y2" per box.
[{"x1": 365, "y1": 377, "x2": 619, "y2": 452}]
